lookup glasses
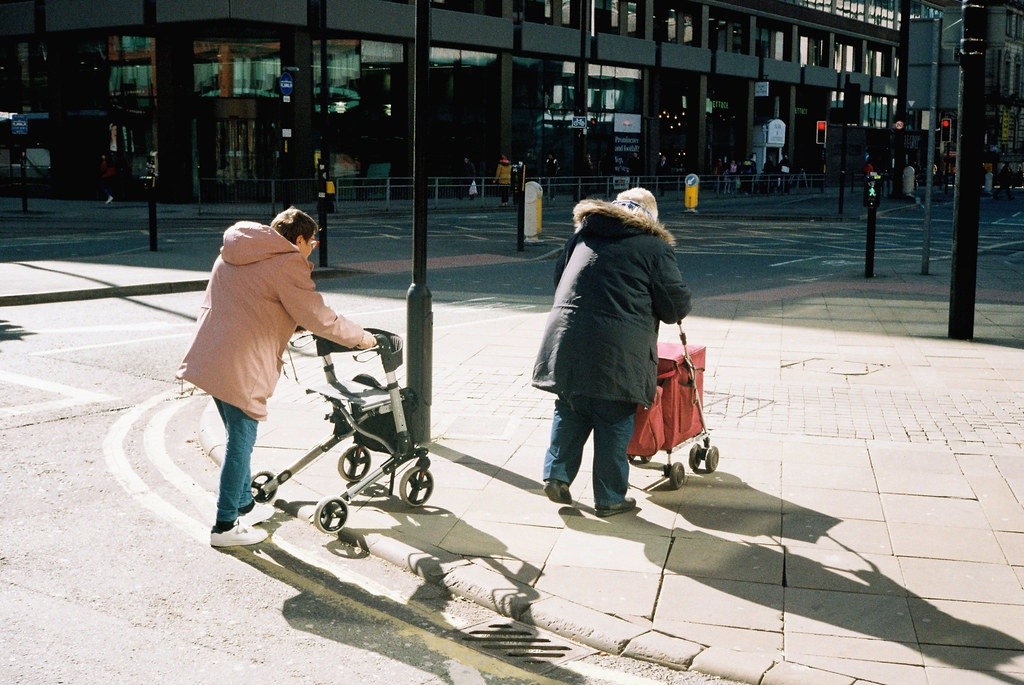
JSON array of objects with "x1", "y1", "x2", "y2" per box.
[{"x1": 302, "y1": 238, "x2": 319, "y2": 248}]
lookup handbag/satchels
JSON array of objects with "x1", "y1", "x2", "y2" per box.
[
  {"x1": 469, "y1": 180, "x2": 478, "y2": 195},
  {"x1": 627, "y1": 385, "x2": 665, "y2": 457}
]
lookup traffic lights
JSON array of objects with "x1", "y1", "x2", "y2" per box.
[
  {"x1": 816, "y1": 120, "x2": 827, "y2": 144},
  {"x1": 941, "y1": 118, "x2": 951, "y2": 142}
]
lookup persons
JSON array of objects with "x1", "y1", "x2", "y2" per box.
[
  {"x1": 655, "y1": 154, "x2": 671, "y2": 197},
  {"x1": 629, "y1": 153, "x2": 639, "y2": 189},
  {"x1": 175, "y1": 208, "x2": 377, "y2": 546},
  {"x1": 100, "y1": 151, "x2": 116, "y2": 204},
  {"x1": 865, "y1": 162, "x2": 873, "y2": 173},
  {"x1": 545, "y1": 153, "x2": 559, "y2": 200},
  {"x1": 993, "y1": 161, "x2": 1015, "y2": 201},
  {"x1": 583, "y1": 151, "x2": 615, "y2": 192},
  {"x1": 913, "y1": 160, "x2": 921, "y2": 190},
  {"x1": 532, "y1": 187, "x2": 691, "y2": 517},
  {"x1": 458, "y1": 155, "x2": 475, "y2": 200},
  {"x1": 493, "y1": 151, "x2": 512, "y2": 206},
  {"x1": 715, "y1": 153, "x2": 791, "y2": 195}
]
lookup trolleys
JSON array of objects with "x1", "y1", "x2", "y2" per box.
[
  {"x1": 625, "y1": 314, "x2": 719, "y2": 489},
  {"x1": 247, "y1": 325, "x2": 434, "y2": 534}
]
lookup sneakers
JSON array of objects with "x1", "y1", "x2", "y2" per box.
[
  {"x1": 594, "y1": 498, "x2": 636, "y2": 517},
  {"x1": 235, "y1": 499, "x2": 275, "y2": 526},
  {"x1": 210, "y1": 520, "x2": 268, "y2": 545},
  {"x1": 544, "y1": 480, "x2": 571, "y2": 505}
]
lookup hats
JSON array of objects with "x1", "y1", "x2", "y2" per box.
[{"x1": 500, "y1": 155, "x2": 510, "y2": 163}]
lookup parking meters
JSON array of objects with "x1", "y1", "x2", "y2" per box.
[
  {"x1": 863, "y1": 172, "x2": 882, "y2": 275},
  {"x1": 138, "y1": 151, "x2": 159, "y2": 250},
  {"x1": 511, "y1": 163, "x2": 525, "y2": 251}
]
